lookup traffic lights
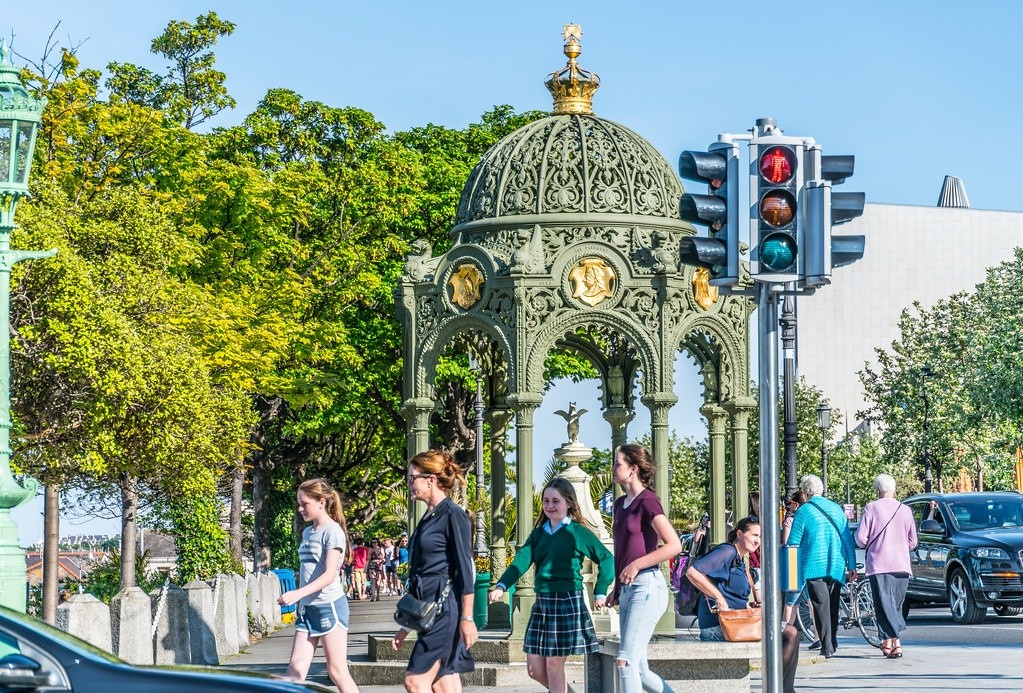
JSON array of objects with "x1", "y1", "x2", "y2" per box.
[
  {"x1": 812, "y1": 145, "x2": 866, "y2": 269},
  {"x1": 680, "y1": 142, "x2": 737, "y2": 284},
  {"x1": 750, "y1": 138, "x2": 805, "y2": 282}
]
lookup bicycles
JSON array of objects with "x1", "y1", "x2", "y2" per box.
[{"x1": 797, "y1": 563, "x2": 884, "y2": 649}]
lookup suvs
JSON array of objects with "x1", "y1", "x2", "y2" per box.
[{"x1": 900, "y1": 492, "x2": 1023, "y2": 624}]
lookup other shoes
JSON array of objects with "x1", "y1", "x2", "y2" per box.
[
  {"x1": 809, "y1": 640, "x2": 822, "y2": 649},
  {"x1": 346, "y1": 592, "x2": 351, "y2": 599},
  {"x1": 370, "y1": 597, "x2": 374, "y2": 602},
  {"x1": 825, "y1": 651, "x2": 831, "y2": 658}
]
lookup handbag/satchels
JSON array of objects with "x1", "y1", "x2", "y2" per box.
[
  {"x1": 718, "y1": 607, "x2": 762, "y2": 641},
  {"x1": 393, "y1": 593, "x2": 437, "y2": 630}
]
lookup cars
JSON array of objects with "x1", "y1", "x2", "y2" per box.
[
  {"x1": 847, "y1": 523, "x2": 867, "y2": 580},
  {"x1": 673, "y1": 534, "x2": 695, "y2": 575},
  {"x1": 0, "y1": 604, "x2": 336, "y2": 693}
]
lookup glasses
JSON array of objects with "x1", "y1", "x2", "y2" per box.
[
  {"x1": 740, "y1": 515, "x2": 758, "y2": 531},
  {"x1": 406, "y1": 473, "x2": 431, "y2": 484},
  {"x1": 788, "y1": 500, "x2": 798, "y2": 507},
  {"x1": 371, "y1": 541, "x2": 378, "y2": 543}
]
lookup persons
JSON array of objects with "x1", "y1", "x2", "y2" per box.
[
  {"x1": 275, "y1": 478, "x2": 359, "y2": 693},
  {"x1": 782, "y1": 492, "x2": 822, "y2": 650},
  {"x1": 489, "y1": 479, "x2": 614, "y2": 693},
  {"x1": 391, "y1": 448, "x2": 479, "y2": 693},
  {"x1": 854, "y1": 474, "x2": 918, "y2": 658},
  {"x1": 787, "y1": 475, "x2": 857, "y2": 658},
  {"x1": 605, "y1": 445, "x2": 683, "y2": 693},
  {"x1": 340, "y1": 532, "x2": 409, "y2": 602},
  {"x1": 927, "y1": 500, "x2": 935, "y2": 520},
  {"x1": 686, "y1": 518, "x2": 799, "y2": 693}
]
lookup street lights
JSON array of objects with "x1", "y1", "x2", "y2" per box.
[
  {"x1": 816, "y1": 399, "x2": 834, "y2": 494},
  {"x1": 919, "y1": 362, "x2": 934, "y2": 493},
  {"x1": 0, "y1": 37, "x2": 47, "y2": 609},
  {"x1": 466, "y1": 343, "x2": 492, "y2": 556}
]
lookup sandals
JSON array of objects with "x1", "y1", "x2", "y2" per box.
[
  {"x1": 886, "y1": 647, "x2": 902, "y2": 659},
  {"x1": 880, "y1": 643, "x2": 892, "y2": 656}
]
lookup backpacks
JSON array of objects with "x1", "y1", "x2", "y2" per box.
[{"x1": 672, "y1": 542, "x2": 736, "y2": 615}]
